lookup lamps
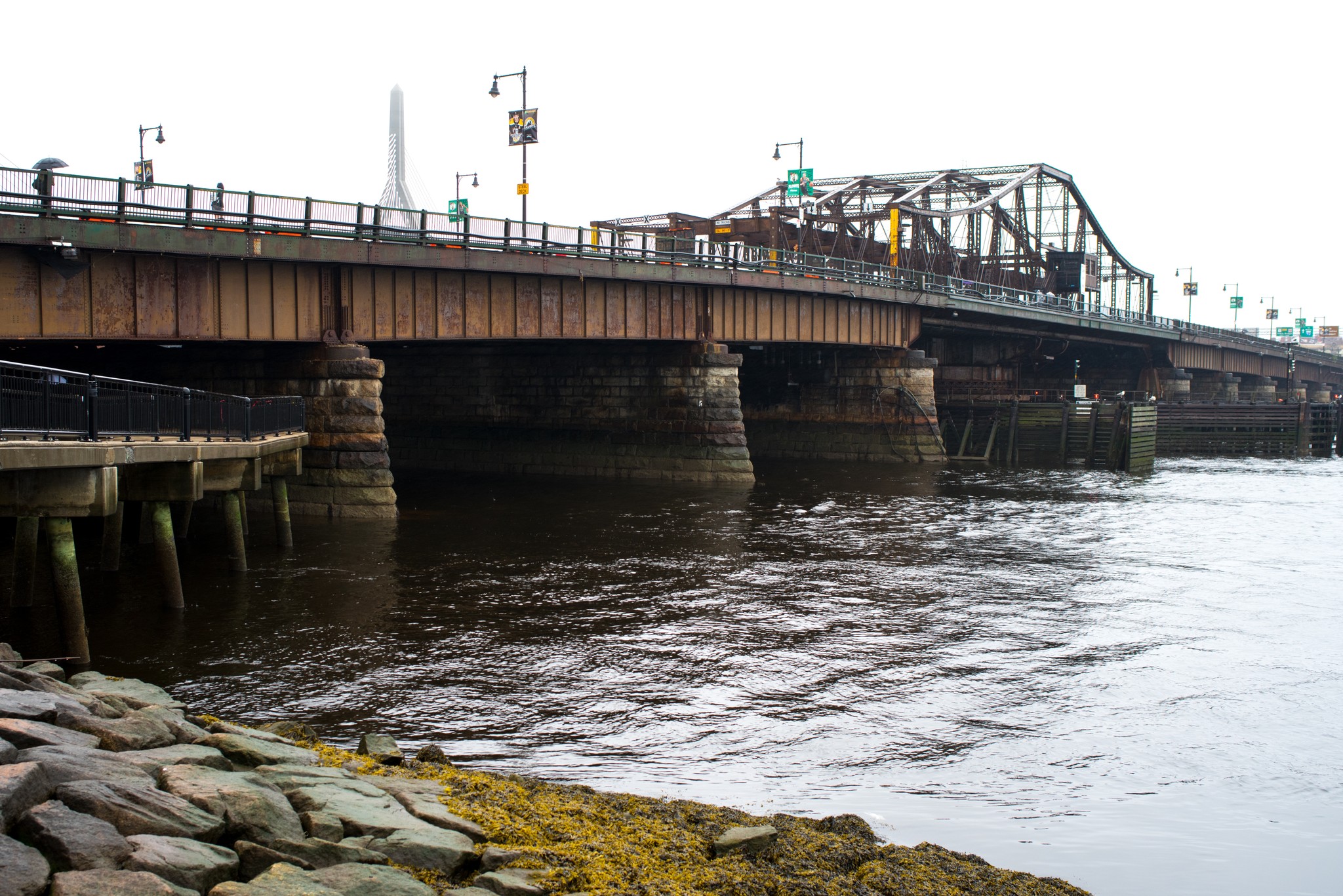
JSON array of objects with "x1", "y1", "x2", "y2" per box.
[
  {"x1": 61, "y1": 247, "x2": 78, "y2": 259},
  {"x1": 952, "y1": 309, "x2": 958, "y2": 317}
]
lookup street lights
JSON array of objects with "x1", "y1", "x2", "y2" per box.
[
  {"x1": 772, "y1": 137, "x2": 803, "y2": 276},
  {"x1": 456, "y1": 170, "x2": 479, "y2": 239},
  {"x1": 1223, "y1": 282, "x2": 1238, "y2": 332},
  {"x1": 1289, "y1": 306, "x2": 1302, "y2": 347},
  {"x1": 489, "y1": 65, "x2": 530, "y2": 245},
  {"x1": 1260, "y1": 295, "x2": 1274, "y2": 340},
  {"x1": 1175, "y1": 266, "x2": 1192, "y2": 329},
  {"x1": 139, "y1": 123, "x2": 166, "y2": 204},
  {"x1": 1313, "y1": 315, "x2": 1325, "y2": 352}
]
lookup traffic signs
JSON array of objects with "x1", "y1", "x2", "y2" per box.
[{"x1": 1300, "y1": 326, "x2": 1313, "y2": 338}]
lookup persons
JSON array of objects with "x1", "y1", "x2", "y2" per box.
[
  {"x1": 1184, "y1": 283, "x2": 1191, "y2": 295},
  {"x1": 800, "y1": 173, "x2": 813, "y2": 196},
  {"x1": 509, "y1": 111, "x2": 524, "y2": 145},
  {"x1": 459, "y1": 202, "x2": 468, "y2": 214},
  {"x1": 1267, "y1": 310, "x2": 1272, "y2": 319},
  {"x1": 135, "y1": 164, "x2": 141, "y2": 190},
  {"x1": 38, "y1": 169, "x2": 55, "y2": 217},
  {"x1": 1236, "y1": 298, "x2": 1242, "y2": 308},
  {"x1": 211, "y1": 183, "x2": 225, "y2": 221}
]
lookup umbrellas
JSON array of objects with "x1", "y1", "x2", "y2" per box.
[{"x1": 32, "y1": 155, "x2": 69, "y2": 170}]
